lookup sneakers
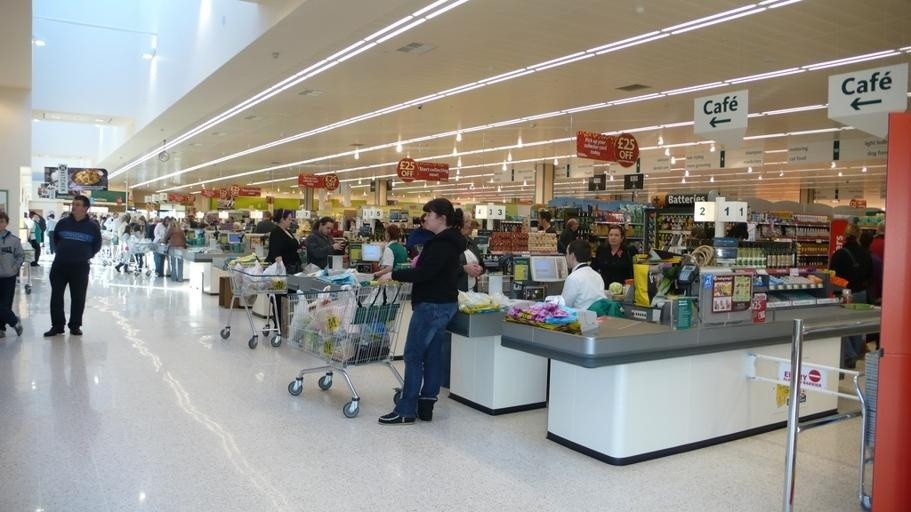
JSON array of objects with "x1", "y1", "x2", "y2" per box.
[
  {"x1": 0, "y1": 329, "x2": 6, "y2": 338},
  {"x1": 68, "y1": 322, "x2": 82, "y2": 335},
  {"x1": 14, "y1": 316, "x2": 23, "y2": 336},
  {"x1": 44, "y1": 327, "x2": 64, "y2": 336}
]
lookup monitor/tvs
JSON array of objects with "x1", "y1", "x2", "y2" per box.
[
  {"x1": 528, "y1": 254, "x2": 569, "y2": 283},
  {"x1": 228, "y1": 233, "x2": 241, "y2": 243},
  {"x1": 362, "y1": 242, "x2": 381, "y2": 262}
]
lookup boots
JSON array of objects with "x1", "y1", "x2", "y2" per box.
[
  {"x1": 379, "y1": 412, "x2": 416, "y2": 424},
  {"x1": 417, "y1": 396, "x2": 437, "y2": 422}
]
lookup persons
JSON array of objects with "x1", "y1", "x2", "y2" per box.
[
  {"x1": 441, "y1": 210, "x2": 487, "y2": 388},
  {"x1": 536, "y1": 211, "x2": 557, "y2": 233},
  {"x1": 1, "y1": 210, "x2": 27, "y2": 337},
  {"x1": 561, "y1": 240, "x2": 607, "y2": 310},
  {"x1": 25, "y1": 208, "x2": 439, "y2": 330},
  {"x1": 377, "y1": 198, "x2": 468, "y2": 425},
  {"x1": 558, "y1": 217, "x2": 581, "y2": 254},
  {"x1": 590, "y1": 225, "x2": 634, "y2": 290},
  {"x1": 828, "y1": 222, "x2": 887, "y2": 369},
  {"x1": 44, "y1": 195, "x2": 103, "y2": 337}
]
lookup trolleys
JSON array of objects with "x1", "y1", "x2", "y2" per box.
[
  {"x1": 12, "y1": 226, "x2": 37, "y2": 294},
  {"x1": 285, "y1": 281, "x2": 405, "y2": 419},
  {"x1": 87, "y1": 228, "x2": 155, "y2": 277},
  {"x1": 220, "y1": 254, "x2": 287, "y2": 348},
  {"x1": 852, "y1": 373, "x2": 874, "y2": 511}
]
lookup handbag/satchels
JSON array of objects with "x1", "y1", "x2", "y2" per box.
[
  {"x1": 39, "y1": 217, "x2": 46, "y2": 231},
  {"x1": 838, "y1": 247, "x2": 874, "y2": 294}
]
